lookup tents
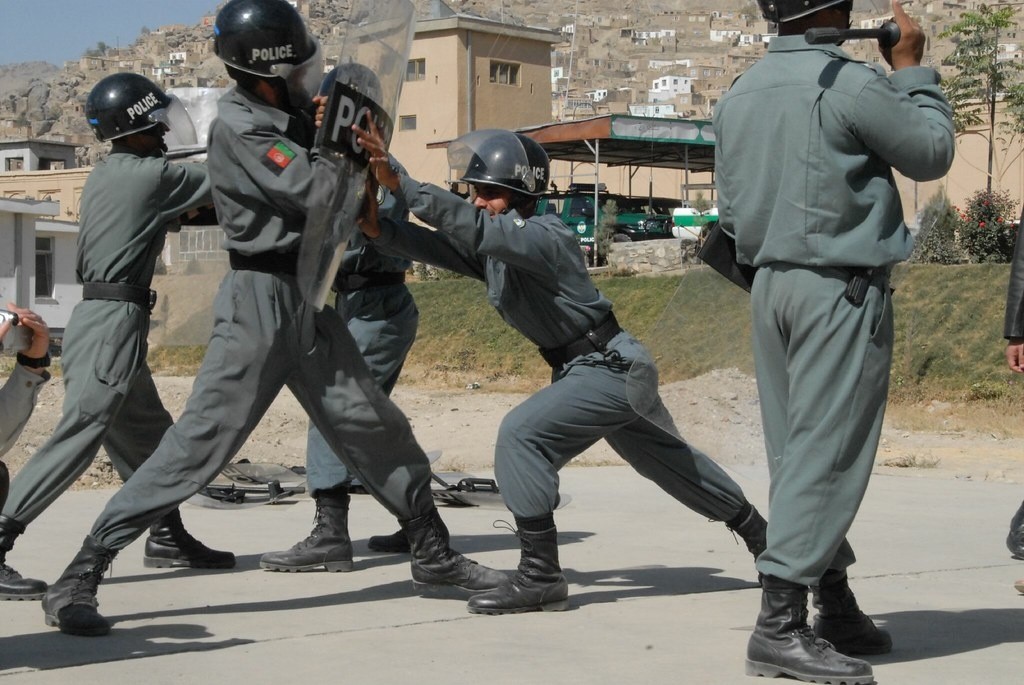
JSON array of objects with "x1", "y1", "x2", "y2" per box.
[{"x1": 427, "y1": 113, "x2": 718, "y2": 267}]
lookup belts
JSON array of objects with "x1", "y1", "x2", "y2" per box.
[
  {"x1": 229, "y1": 250, "x2": 298, "y2": 274},
  {"x1": 82, "y1": 281, "x2": 150, "y2": 303},
  {"x1": 539, "y1": 311, "x2": 620, "y2": 369},
  {"x1": 334, "y1": 272, "x2": 406, "y2": 294}
]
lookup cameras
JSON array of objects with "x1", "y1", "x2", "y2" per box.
[{"x1": 0, "y1": 308, "x2": 33, "y2": 350}]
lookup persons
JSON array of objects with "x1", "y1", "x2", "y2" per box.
[
  {"x1": 1004, "y1": 201, "x2": 1024, "y2": 560},
  {"x1": 259, "y1": 63, "x2": 450, "y2": 572},
  {"x1": 0, "y1": 302, "x2": 51, "y2": 515},
  {"x1": 0, "y1": 72, "x2": 236, "y2": 601},
  {"x1": 42, "y1": 0, "x2": 509, "y2": 637},
  {"x1": 351, "y1": 110, "x2": 769, "y2": 615},
  {"x1": 713, "y1": 0, "x2": 955, "y2": 685}
]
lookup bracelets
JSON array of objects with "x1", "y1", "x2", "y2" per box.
[{"x1": 17, "y1": 351, "x2": 52, "y2": 368}]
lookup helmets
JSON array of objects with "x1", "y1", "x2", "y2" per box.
[
  {"x1": 757, "y1": 0, "x2": 844, "y2": 23},
  {"x1": 213, "y1": 0, "x2": 318, "y2": 77},
  {"x1": 460, "y1": 132, "x2": 549, "y2": 196},
  {"x1": 319, "y1": 62, "x2": 383, "y2": 105},
  {"x1": 85, "y1": 72, "x2": 172, "y2": 142}
]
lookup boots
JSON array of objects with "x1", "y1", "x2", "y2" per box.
[
  {"x1": 726, "y1": 505, "x2": 768, "y2": 560},
  {"x1": 812, "y1": 576, "x2": 892, "y2": 654},
  {"x1": 41, "y1": 535, "x2": 119, "y2": 636},
  {"x1": 368, "y1": 528, "x2": 411, "y2": 554},
  {"x1": 144, "y1": 507, "x2": 235, "y2": 569},
  {"x1": 467, "y1": 519, "x2": 569, "y2": 615},
  {"x1": 398, "y1": 506, "x2": 508, "y2": 597},
  {"x1": 0, "y1": 514, "x2": 47, "y2": 600},
  {"x1": 744, "y1": 573, "x2": 874, "y2": 685},
  {"x1": 260, "y1": 496, "x2": 352, "y2": 573}
]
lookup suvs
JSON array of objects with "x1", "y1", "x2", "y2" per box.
[{"x1": 536, "y1": 182, "x2": 673, "y2": 267}]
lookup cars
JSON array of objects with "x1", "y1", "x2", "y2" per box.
[{"x1": 672, "y1": 207, "x2": 719, "y2": 241}]
